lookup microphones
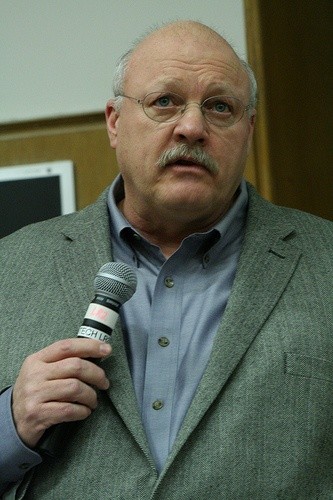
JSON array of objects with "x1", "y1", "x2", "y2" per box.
[{"x1": 35, "y1": 262, "x2": 138, "y2": 463}]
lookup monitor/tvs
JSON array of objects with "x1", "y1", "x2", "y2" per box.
[{"x1": 1, "y1": 158, "x2": 77, "y2": 238}]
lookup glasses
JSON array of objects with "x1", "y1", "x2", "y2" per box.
[{"x1": 116, "y1": 89, "x2": 255, "y2": 128}]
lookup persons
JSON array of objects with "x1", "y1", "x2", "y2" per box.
[{"x1": 0, "y1": 14, "x2": 332, "y2": 500}]
